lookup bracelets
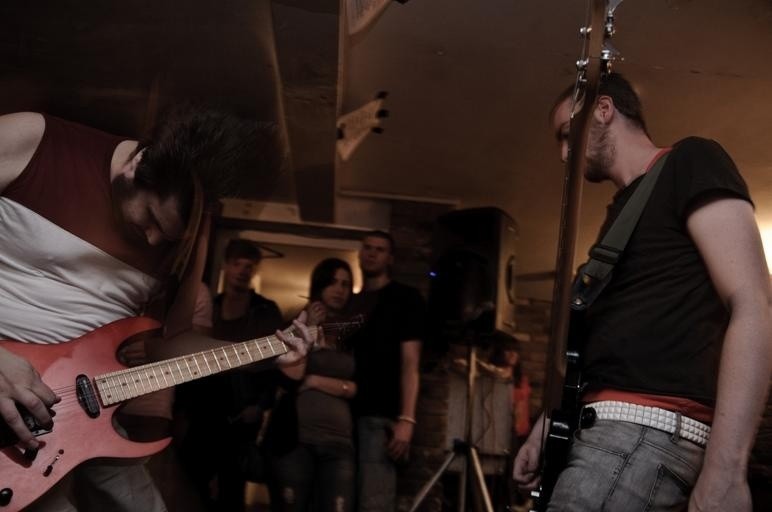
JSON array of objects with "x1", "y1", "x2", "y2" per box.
[
  {"x1": 340, "y1": 378, "x2": 349, "y2": 398},
  {"x1": 396, "y1": 414, "x2": 418, "y2": 425}
]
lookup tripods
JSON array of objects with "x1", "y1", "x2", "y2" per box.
[{"x1": 404, "y1": 374, "x2": 497, "y2": 512}]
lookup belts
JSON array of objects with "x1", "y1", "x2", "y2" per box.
[{"x1": 583, "y1": 400, "x2": 711, "y2": 447}]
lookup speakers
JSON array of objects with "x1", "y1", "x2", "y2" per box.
[{"x1": 426, "y1": 205, "x2": 521, "y2": 346}]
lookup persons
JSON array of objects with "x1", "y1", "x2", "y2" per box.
[
  {"x1": 488, "y1": 333, "x2": 533, "y2": 437},
  {"x1": 509, "y1": 69, "x2": 770, "y2": 512},
  {"x1": 169, "y1": 238, "x2": 287, "y2": 512},
  {"x1": 335, "y1": 229, "x2": 429, "y2": 512},
  {"x1": 256, "y1": 254, "x2": 357, "y2": 511},
  {"x1": 1, "y1": 103, "x2": 325, "y2": 512}
]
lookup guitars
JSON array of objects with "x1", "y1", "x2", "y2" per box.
[
  {"x1": 507, "y1": 2, "x2": 621, "y2": 511},
  {"x1": 0, "y1": 311, "x2": 364, "y2": 510}
]
